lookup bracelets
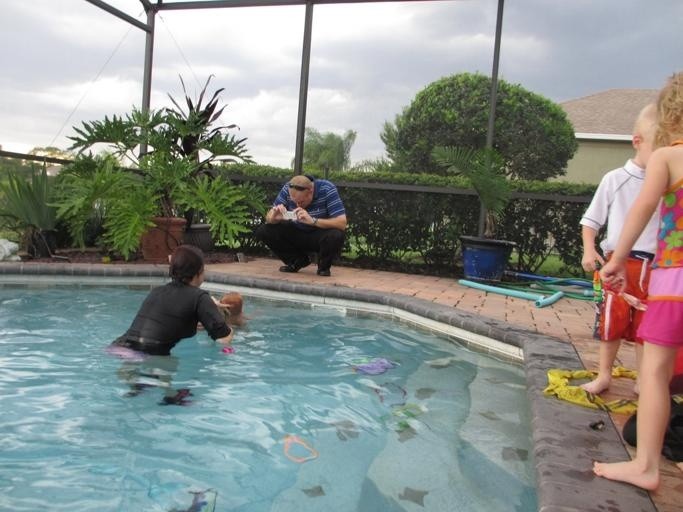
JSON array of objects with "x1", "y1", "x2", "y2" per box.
[{"x1": 312, "y1": 217, "x2": 317, "y2": 225}]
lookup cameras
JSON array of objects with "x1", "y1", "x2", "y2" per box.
[{"x1": 283, "y1": 211, "x2": 298, "y2": 220}]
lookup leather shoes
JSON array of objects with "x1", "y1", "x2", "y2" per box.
[
  {"x1": 279, "y1": 254, "x2": 311, "y2": 272},
  {"x1": 317, "y1": 260, "x2": 330, "y2": 275}
]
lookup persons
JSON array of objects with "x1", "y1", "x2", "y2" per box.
[
  {"x1": 601, "y1": 69, "x2": 683, "y2": 491},
  {"x1": 256, "y1": 174, "x2": 347, "y2": 276},
  {"x1": 107, "y1": 245, "x2": 244, "y2": 405},
  {"x1": 581, "y1": 103, "x2": 663, "y2": 394}
]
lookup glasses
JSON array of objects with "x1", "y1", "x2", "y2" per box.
[{"x1": 288, "y1": 183, "x2": 309, "y2": 190}]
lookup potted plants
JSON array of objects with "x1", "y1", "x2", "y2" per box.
[
  {"x1": 0, "y1": 90, "x2": 268, "y2": 265},
  {"x1": 434, "y1": 143, "x2": 527, "y2": 287}
]
